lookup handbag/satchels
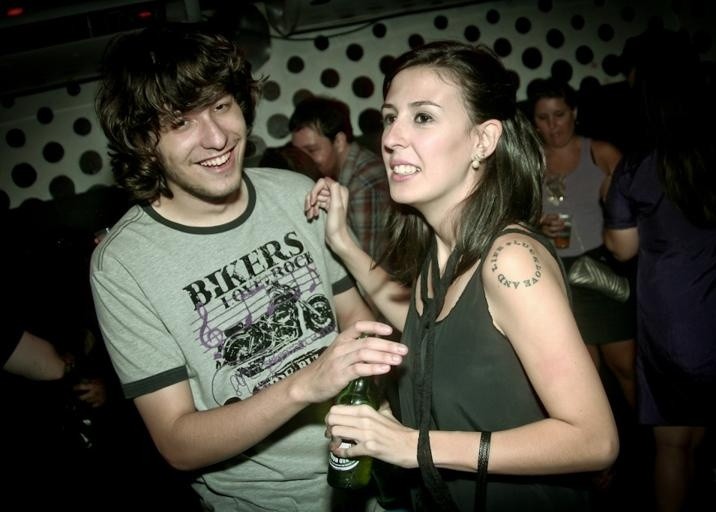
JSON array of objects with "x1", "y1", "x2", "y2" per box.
[{"x1": 569, "y1": 256, "x2": 630, "y2": 302}]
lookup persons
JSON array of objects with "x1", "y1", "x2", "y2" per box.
[
  {"x1": 2, "y1": 185, "x2": 202, "y2": 512},
  {"x1": 260, "y1": 97, "x2": 393, "y2": 271},
  {"x1": 526, "y1": 32, "x2": 714, "y2": 512},
  {"x1": 304, "y1": 40, "x2": 620, "y2": 512},
  {"x1": 89, "y1": 29, "x2": 409, "y2": 512}
]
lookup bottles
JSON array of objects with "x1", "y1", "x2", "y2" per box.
[
  {"x1": 326, "y1": 327, "x2": 383, "y2": 492},
  {"x1": 553, "y1": 210, "x2": 571, "y2": 249}
]
